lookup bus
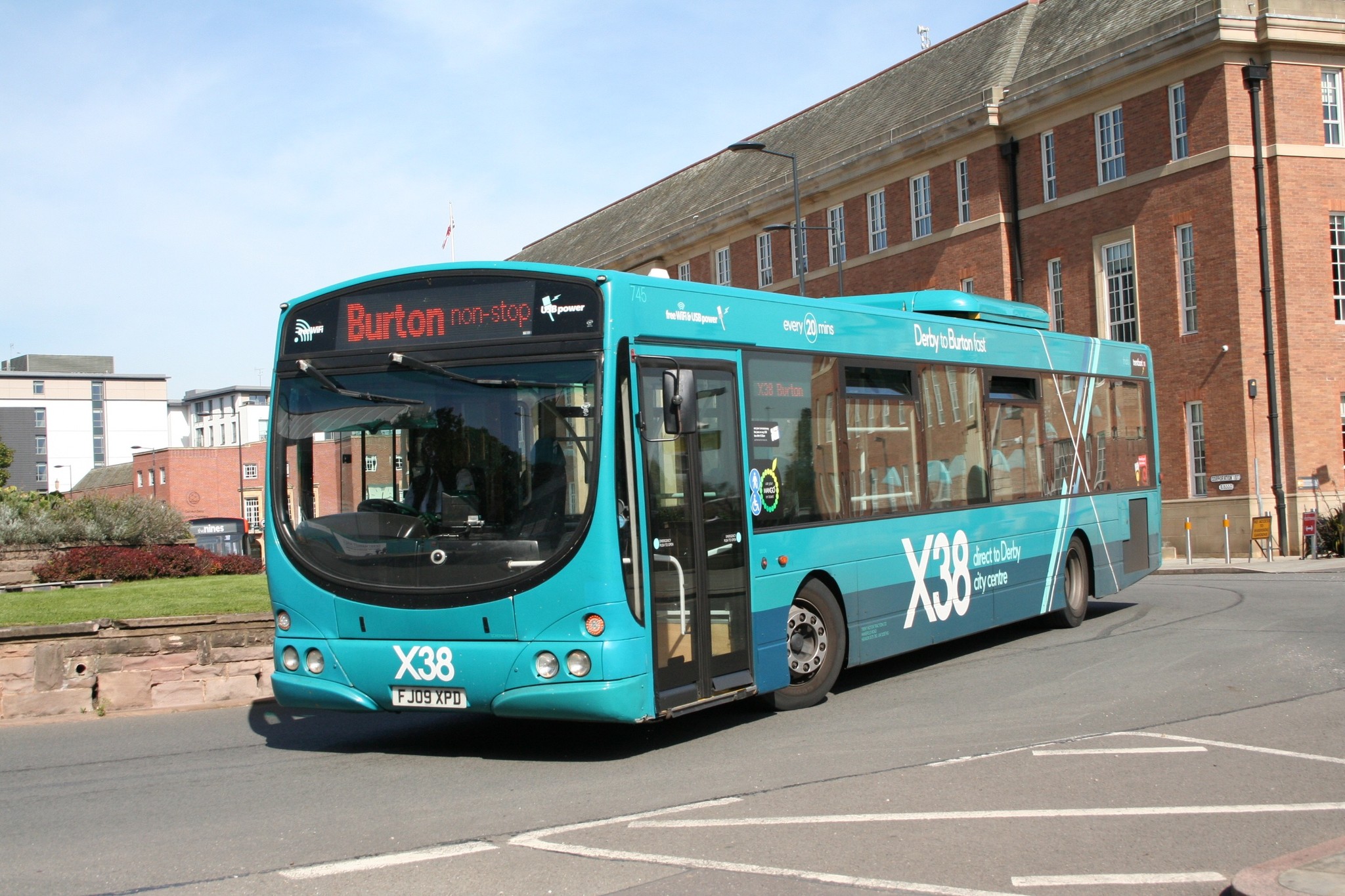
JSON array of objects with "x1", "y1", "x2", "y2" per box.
[
  {"x1": 262, "y1": 259, "x2": 1161, "y2": 725},
  {"x1": 186, "y1": 517, "x2": 256, "y2": 560}
]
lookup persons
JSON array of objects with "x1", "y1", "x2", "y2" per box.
[{"x1": 402, "y1": 434, "x2": 476, "y2": 537}]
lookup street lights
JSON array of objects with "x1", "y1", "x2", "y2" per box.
[
  {"x1": 54, "y1": 465, "x2": 72, "y2": 500},
  {"x1": 131, "y1": 445, "x2": 156, "y2": 499},
  {"x1": 728, "y1": 142, "x2": 805, "y2": 296},
  {"x1": 762, "y1": 224, "x2": 843, "y2": 298},
  {"x1": 198, "y1": 411, "x2": 244, "y2": 519}
]
